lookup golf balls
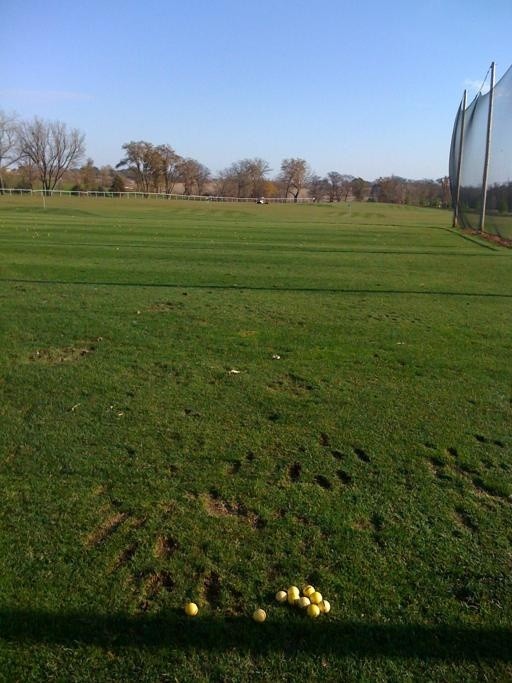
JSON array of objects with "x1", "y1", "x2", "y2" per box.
[
  {"x1": 253, "y1": 608, "x2": 266, "y2": 623},
  {"x1": 276, "y1": 584, "x2": 331, "y2": 619},
  {"x1": 184, "y1": 602, "x2": 198, "y2": 616}
]
